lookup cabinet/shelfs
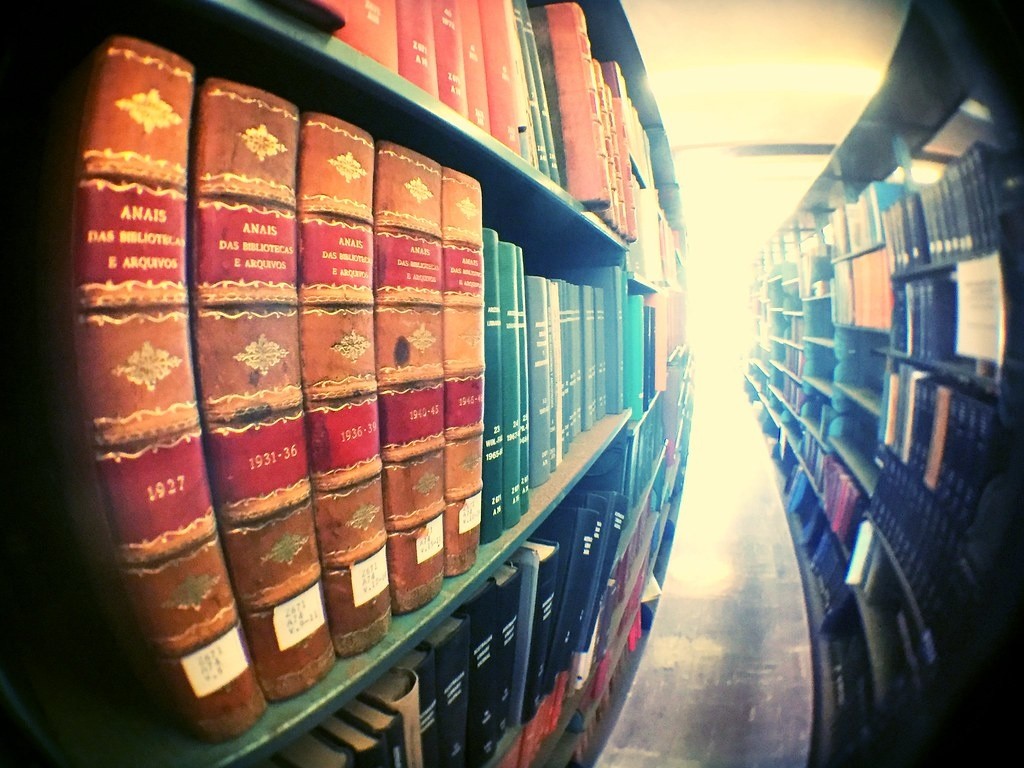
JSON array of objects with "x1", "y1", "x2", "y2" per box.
[
  {"x1": 739, "y1": 0, "x2": 1024, "y2": 768},
  {"x1": 80, "y1": 0, "x2": 695, "y2": 768}
]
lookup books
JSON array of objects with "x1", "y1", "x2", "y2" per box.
[
  {"x1": 743, "y1": 143, "x2": 1022, "y2": 767},
  {"x1": 77, "y1": 1, "x2": 695, "y2": 768}
]
